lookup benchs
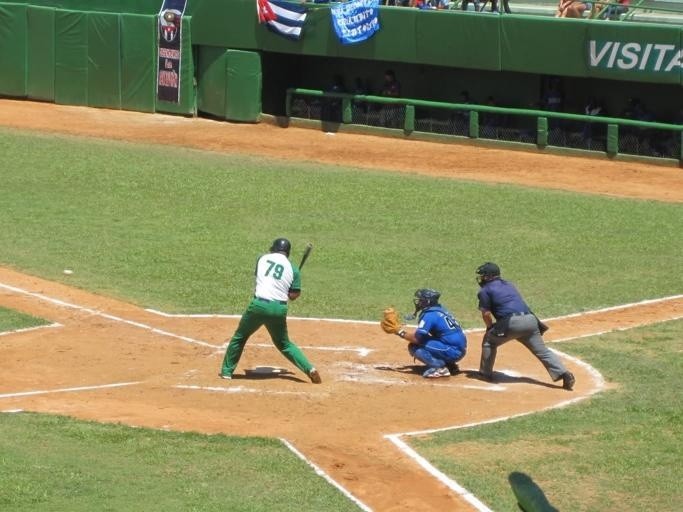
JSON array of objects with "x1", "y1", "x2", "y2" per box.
[{"x1": 447, "y1": 2, "x2": 683, "y2": 24}]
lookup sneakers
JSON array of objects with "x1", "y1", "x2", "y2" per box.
[
  {"x1": 471, "y1": 370, "x2": 492, "y2": 383},
  {"x1": 562, "y1": 373, "x2": 576, "y2": 388},
  {"x1": 427, "y1": 364, "x2": 459, "y2": 378},
  {"x1": 309, "y1": 366, "x2": 321, "y2": 384}
]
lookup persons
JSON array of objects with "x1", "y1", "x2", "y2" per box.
[
  {"x1": 349, "y1": 74, "x2": 371, "y2": 111},
  {"x1": 323, "y1": 75, "x2": 345, "y2": 106},
  {"x1": 380, "y1": 0, "x2": 637, "y2": 22},
  {"x1": 475, "y1": 262, "x2": 575, "y2": 390},
  {"x1": 381, "y1": 287, "x2": 467, "y2": 378},
  {"x1": 446, "y1": 91, "x2": 482, "y2": 128},
  {"x1": 527, "y1": 75, "x2": 564, "y2": 130},
  {"x1": 222, "y1": 238, "x2": 321, "y2": 384},
  {"x1": 377, "y1": 71, "x2": 403, "y2": 127},
  {"x1": 574, "y1": 94, "x2": 661, "y2": 156}
]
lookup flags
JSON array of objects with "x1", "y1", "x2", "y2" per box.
[
  {"x1": 330, "y1": 1, "x2": 382, "y2": 46},
  {"x1": 252, "y1": 1, "x2": 308, "y2": 41}
]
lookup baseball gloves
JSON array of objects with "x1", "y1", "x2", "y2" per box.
[{"x1": 381, "y1": 309, "x2": 402, "y2": 334}]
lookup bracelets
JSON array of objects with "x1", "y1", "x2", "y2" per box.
[{"x1": 398, "y1": 329, "x2": 406, "y2": 337}]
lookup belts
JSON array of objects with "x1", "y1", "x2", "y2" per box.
[
  {"x1": 259, "y1": 298, "x2": 287, "y2": 305},
  {"x1": 509, "y1": 311, "x2": 532, "y2": 315}
]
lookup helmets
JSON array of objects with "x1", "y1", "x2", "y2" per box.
[
  {"x1": 413, "y1": 287, "x2": 441, "y2": 313},
  {"x1": 475, "y1": 262, "x2": 500, "y2": 287},
  {"x1": 270, "y1": 239, "x2": 290, "y2": 256}
]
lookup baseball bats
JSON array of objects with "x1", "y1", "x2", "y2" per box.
[{"x1": 298, "y1": 244, "x2": 312, "y2": 271}]
